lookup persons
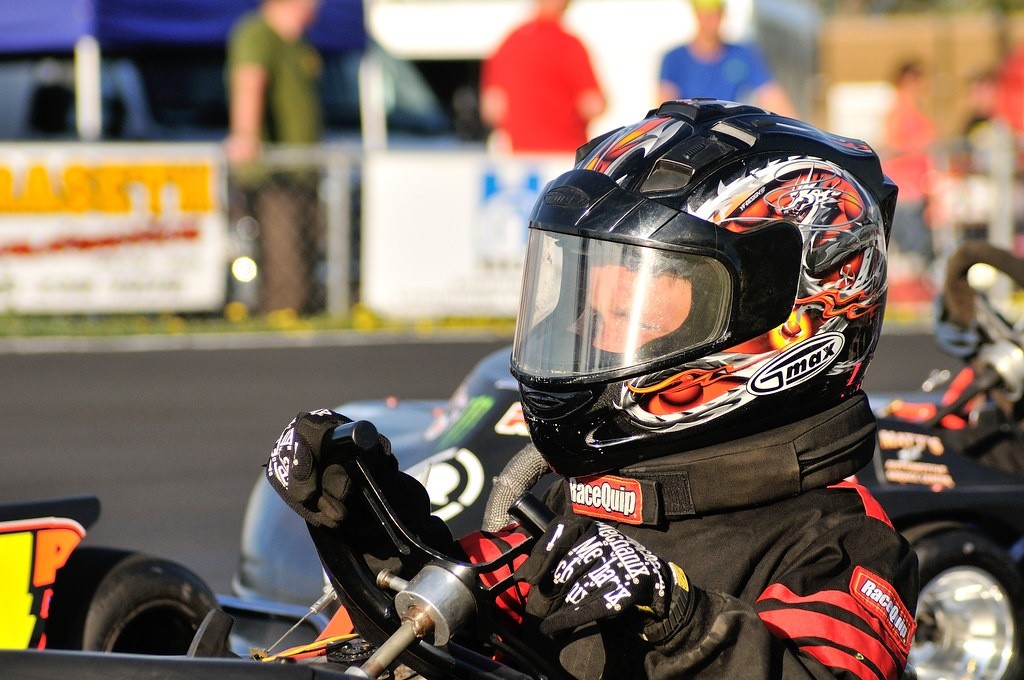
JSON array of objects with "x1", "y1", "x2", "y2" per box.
[
  {"x1": 480, "y1": 0, "x2": 606, "y2": 153},
  {"x1": 881, "y1": 60, "x2": 937, "y2": 160},
  {"x1": 657, "y1": 0, "x2": 797, "y2": 120},
  {"x1": 266, "y1": 99, "x2": 921, "y2": 680},
  {"x1": 219, "y1": 0, "x2": 328, "y2": 320}
]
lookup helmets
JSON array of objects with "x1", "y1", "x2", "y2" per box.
[{"x1": 508, "y1": 99, "x2": 899, "y2": 479}]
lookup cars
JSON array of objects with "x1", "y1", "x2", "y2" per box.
[{"x1": 0, "y1": 28, "x2": 463, "y2": 323}]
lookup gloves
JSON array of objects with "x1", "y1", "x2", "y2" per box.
[
  {"x1": 513, "y1": 516, "x2": 690, "y2": 644},
  {"x1": 260, "y1": 409, "x2": 453, "y2": 578}
]
